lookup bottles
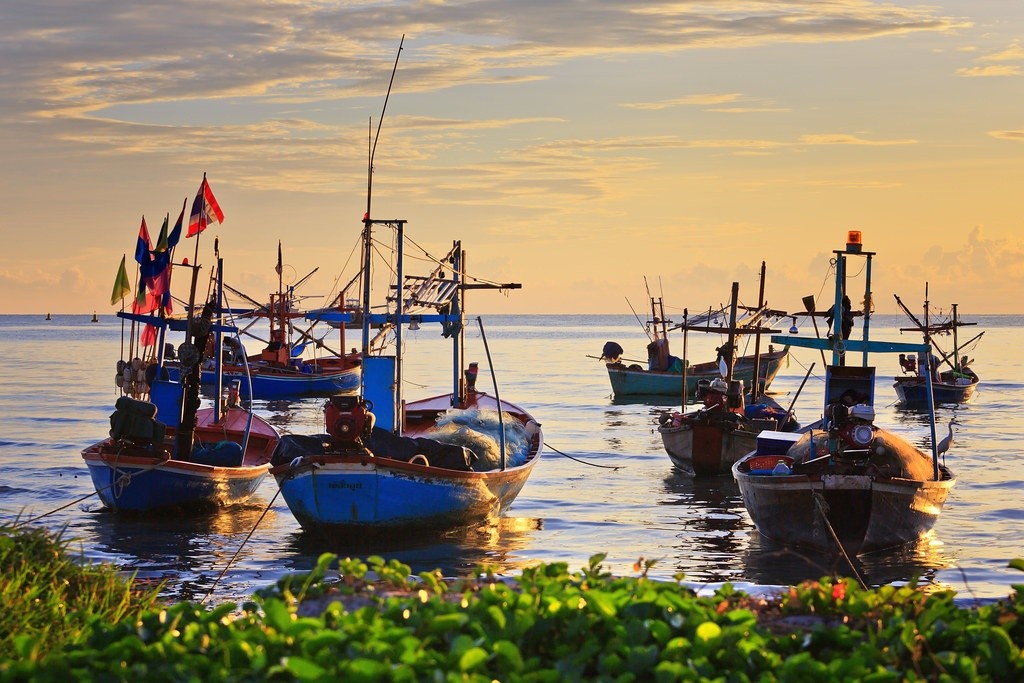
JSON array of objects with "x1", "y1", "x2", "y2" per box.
[{"x1": 772, "y1": 460, "x2": 790, "y2": 476}]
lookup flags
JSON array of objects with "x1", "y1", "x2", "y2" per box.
[
  {"x1": 132, "y1": 209, "x2": 183, "y2": 348},
  {"x1": 188, "y1": 178, "x2": 224, "y2": 238},
  {"x1": 110, "y1": 260, "x2": 130, "y2": 305}
]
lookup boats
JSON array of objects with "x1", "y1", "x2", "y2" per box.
[
  {"x1": 584, "y1": 230, "x2": 985, "y2": 553},
  {"x1": 268, "y1": 29, "x2": 545, "y2": 550},
  {"x1": 81, "y1": 171, "x2": 280, "y2": 516},
  {"x1": 149, "y1": 237, "x2": 362, "y2": 400}
]
